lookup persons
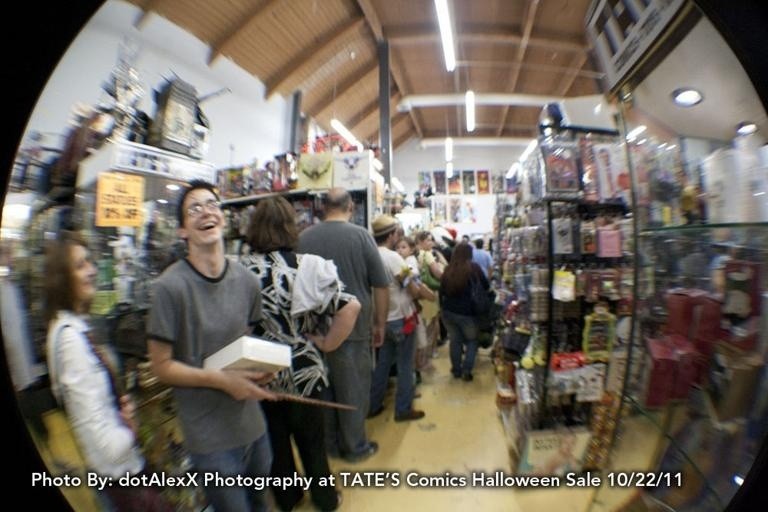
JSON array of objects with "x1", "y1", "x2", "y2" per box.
[
  {"x1": 369, "y1": 214, "x2": 503, "y2": 424},
  {"x1": 40, "y1": 226, "x2": 188, "y2": 511},
  {"x1": 146, "y1": 179, "x2": 279, "y2": 511},
  {"x1": 295, "y1": 186, "x2": 392, "y2": 465},
  {"x1": 230, "y1": 193, "x2": 363, "y2": 512}
]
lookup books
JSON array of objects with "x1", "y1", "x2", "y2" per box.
[{"x1": 202, "y1": 334, "x2": 290, "y2": 375}]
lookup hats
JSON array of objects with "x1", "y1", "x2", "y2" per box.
[{"x1": 372, "y1": 213, "x2": 396, "y2": 237}]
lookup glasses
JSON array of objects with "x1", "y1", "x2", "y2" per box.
[{"x1": 187, "y1": 199, "x2": 221, "y2": 217}]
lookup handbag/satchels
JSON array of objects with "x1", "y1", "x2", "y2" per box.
[
  {"x1": 470, "y1": 272, "x2": 490, "y2": 314},
  {"x1": 419, "y1": 251, "x2": 441, "y2": 290}
]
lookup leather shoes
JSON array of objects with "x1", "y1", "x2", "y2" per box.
[
  {"x1": 335, "y1": 490, "x2": 343, "y2": 510},
  {"x1": 367, "y1": 441, "x2": 378, "y2": 456},
  {"x1": 395, "y1": 410, "x2": 425, "y2": 420},
  {"x1": 460, "y1": 372, "x2": 474, "y2": 381}
]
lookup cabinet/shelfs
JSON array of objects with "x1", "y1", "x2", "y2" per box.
[
  {"x1": 586, "y1": 222, "x2": 768, "y2": 511},
  {"x1": 218, "y1": 189, "x2": 374, "y2": 266}
]
